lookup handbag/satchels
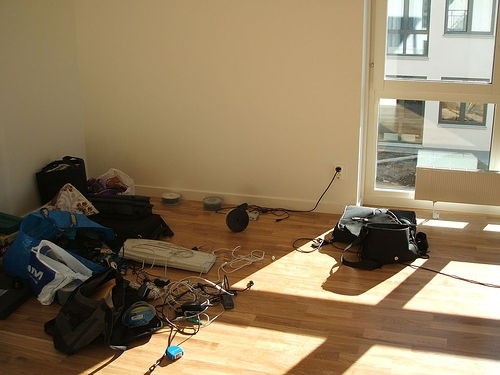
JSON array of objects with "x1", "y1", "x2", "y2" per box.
[
  {"x1": 332, "y1": 205, "x2": 416, "y2": 245},
  {"x1": 2, "y1": 208, "x2": 122, "y2": 282},
  {"x1": 53, "y1": 267, "x2": 126, "y2": 355},
  {"x1": 361, "y1": 223, "x2": 410, "y2": 264},
  {"x1": 23, "y1": 240, "x2": 94, "y2": 306},
  {"x1": 35, "y1": 155, "x2": 88, "y2": 203}
]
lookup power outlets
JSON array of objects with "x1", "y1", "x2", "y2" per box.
[{"x1": 333, "y1": 164, "x2": 345, "y2": 180}]
